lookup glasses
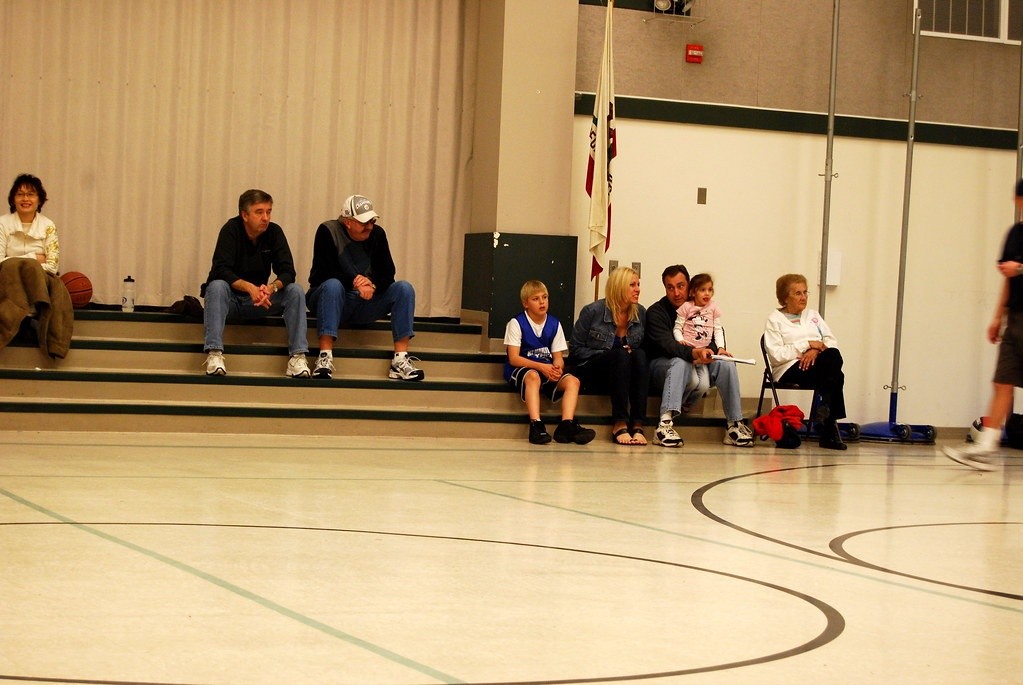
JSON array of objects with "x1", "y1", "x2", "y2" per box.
[
  {"x1": 349, "y1": 219, "x2": 377, "y2": 225},
  {"x1": 15, "y1": 189, "x2": 38, "y2": 198}
]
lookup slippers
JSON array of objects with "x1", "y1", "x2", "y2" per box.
[{"x1": 611, "y1": 429, "x2": 647, "y2": 445}]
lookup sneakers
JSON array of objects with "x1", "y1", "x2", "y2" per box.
[
  {"x1": 721, "y1": 419, "x2": 755, "y2": 447},
  {"x1": 651, "y1": 419, "x2": 684, "y2": 447},
  {"x1": 388, "y1": 355, "x2": 426, "y2": 380},
  {"x1": 528, "y1": 418, "x2": 552, "y2": 443},
  {"x1": 286, "y1": 355, "x2": 312, "y2": 378},
  {"x1": 313, "y1": 357, "x2": 335, "y2": 377},
  {"x1": 553, "y1": 418, "x2": 598, "y2": 444},
  {"x1": 201, "y1": 350, "x2": 227, "y2": 375}
]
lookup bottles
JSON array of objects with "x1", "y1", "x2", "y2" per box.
[{"x1": 122, "y1": 276, "x2": 135, "y2": 313}]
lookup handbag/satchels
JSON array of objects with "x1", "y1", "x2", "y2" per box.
[{"x1": 752, "y1": 403, "x2": 805, "y2": 449}]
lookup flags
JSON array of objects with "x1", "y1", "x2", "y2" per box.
[{"x1": 585, "y1": 5, "x2": 617, "y2": 288}]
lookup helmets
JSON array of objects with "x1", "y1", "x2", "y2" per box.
[{"x1": 969, "y1": 416, "x2": 987, "y2": 443}]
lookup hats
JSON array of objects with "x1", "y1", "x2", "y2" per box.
[{"x1": 341, "y1": 196, "x2": 379, "y2": 223}]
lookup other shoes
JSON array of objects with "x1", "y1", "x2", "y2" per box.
[
  {"x1": 813, "y1": 406, "x2": 847, "y2": 450},
  {"x1": 941, "y1": 446, "x2": 994, "y2": 470}
]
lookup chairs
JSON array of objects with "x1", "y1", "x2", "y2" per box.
[{"x1": 752, "y1": 334, "x2": 818, "y2": 445}]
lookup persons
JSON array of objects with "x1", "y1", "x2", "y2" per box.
[
  {"x1": 942, "y1": 177, "x2": 1023, "y2": 472},
  {"x1": 200, "y1": 189, "x2": 311, "y2": 378},
  {"x1": 563, "y1": 266, "x2": 647, "y2": 445},
  {"x1": 764, "y1": 274, "x2": 848, "y2": 450},
  {"x1": 305, "y1": 195, "x2": 425, "y2": 381},
  {"x1": 644, "y1": 265, "x2": 754, "y2": 447},
  {"x1": 0, "y1": 174, "x2": 60, "y2": 318},
  {"x1": 503, "y1": 279, "x2": 597, "y2": 445}
]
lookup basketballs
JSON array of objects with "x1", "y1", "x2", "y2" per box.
[{"x1": 60, "y1": 270, "x2": 94, "y2": 309}]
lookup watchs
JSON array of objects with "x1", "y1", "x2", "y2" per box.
[
  {"x1": 372, "y1": 283, "x2": 376, "y2": 290},
  {"x1": 271, "y1": 282, "x2": 278, "y2": 294}
]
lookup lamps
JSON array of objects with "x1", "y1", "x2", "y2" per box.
[{"x1": 654, "y1": 0, "x2": 694, "y2": 12}]
